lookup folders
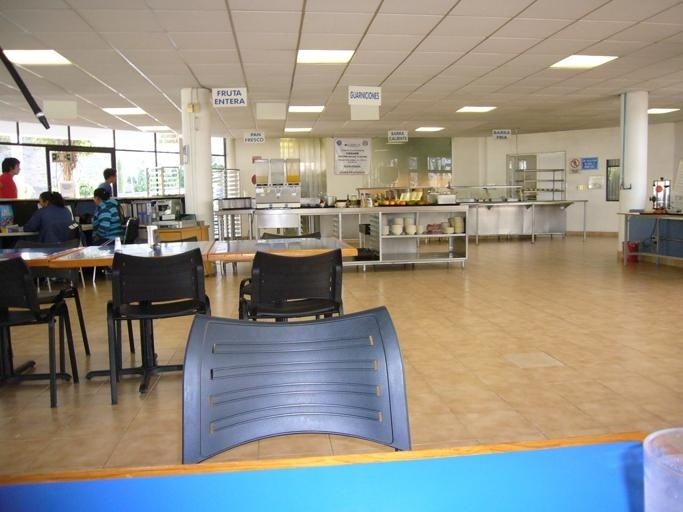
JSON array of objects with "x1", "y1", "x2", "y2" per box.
[{"x1": 133, "y1": 202, "x2": 151, "y2": 226}]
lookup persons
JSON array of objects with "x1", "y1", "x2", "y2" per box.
[
  {"x1": 91, "y1": 188, "x2": 124, "y2": 246},
  {"x1": 98, "y1": 169, "x2": 116, "y2": 198},
  {"x1": 23, "y1": 192, "x2": 72, "y2": 248},
  {"x1": 1, "y1": 157, "x2": 20, "y2": 198}
]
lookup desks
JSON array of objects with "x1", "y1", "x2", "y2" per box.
[
  {"x1": 157, "y1": 225, "x2": 210, "y2": 277},
  {"x1": 1, "y1": 232, "x2": 41, "y2": 239},
  {"x1": 1, "y1": 245, "x2": 83, "y2": 265},
  {"x1": 49, "y1": 237, "x2": 358, "y2": 395}
]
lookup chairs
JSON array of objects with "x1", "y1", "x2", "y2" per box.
[
  {"x1": 36, "y1": 227, "x2": 85, "y2": 294},
  {"x1": 106, "y1": 248, "x2": 211, "y2": 405},
  {"x1": 125, "y1": 217, "x2": 139, "y2": 243},
  {"x1": 0, "y1": 258, "x2": 79, "y2": 407},
  {"x1": 239, "y1": 250, "x2": 343, "y2": 321}
]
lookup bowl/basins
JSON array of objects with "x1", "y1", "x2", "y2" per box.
[
  {"x1": 382, "y1": 217, "x2": 425, "y2": 235},
  {"x1": 440, "y1": 216, "x2": 465, "y2": 234}
]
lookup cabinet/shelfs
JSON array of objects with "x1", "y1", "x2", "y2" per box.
[
  {"x1": 617, "y1": 212, "x2": 683, "y2": 268},
  {"x1": 472, "y1": 201, "x2": 590, "y2": 245},
  {"x1": 515, "y1": 168, "x2": 567, "y2": 198},
  {"x1": 218, "y1": 205, "x2": 469, "y2": 276}
]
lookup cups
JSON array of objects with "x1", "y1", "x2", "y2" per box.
[{"x1": 641, "y1": 429, "x2": 681, "y2": 512}]
